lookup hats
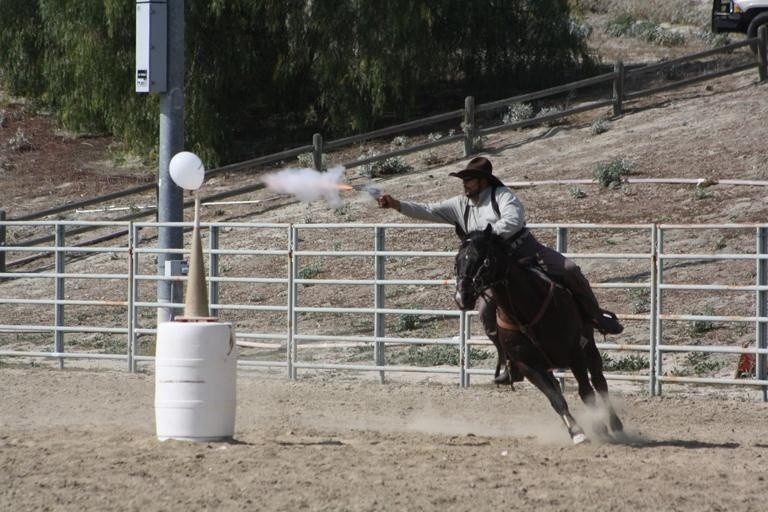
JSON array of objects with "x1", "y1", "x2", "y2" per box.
[{"x1": 448, "y1": 157, "x2": 493, "y2": 181}]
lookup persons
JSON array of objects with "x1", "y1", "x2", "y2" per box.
[{"x1": 376, "y1": 157, "x2": 624, "y2": 384}]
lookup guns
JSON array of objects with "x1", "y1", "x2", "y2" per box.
[{"x1": 352, "y1": 185, "x2": 388, "y2": 209}]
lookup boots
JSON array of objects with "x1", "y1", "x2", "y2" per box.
[
  {"x1": 578, "y1": 296, "x2": 624, "y2": 335},
  {"x1": 488, "y1": 334, "x2": 523, "y2": 385}
]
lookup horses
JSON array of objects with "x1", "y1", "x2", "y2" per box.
[{"x1": 452, "y1": 219, "x2": 628, "y2": 445}]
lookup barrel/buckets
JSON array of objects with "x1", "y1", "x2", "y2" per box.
[{"x1": 152, "y1": 317, "x2": 237, "y2": 445}]
